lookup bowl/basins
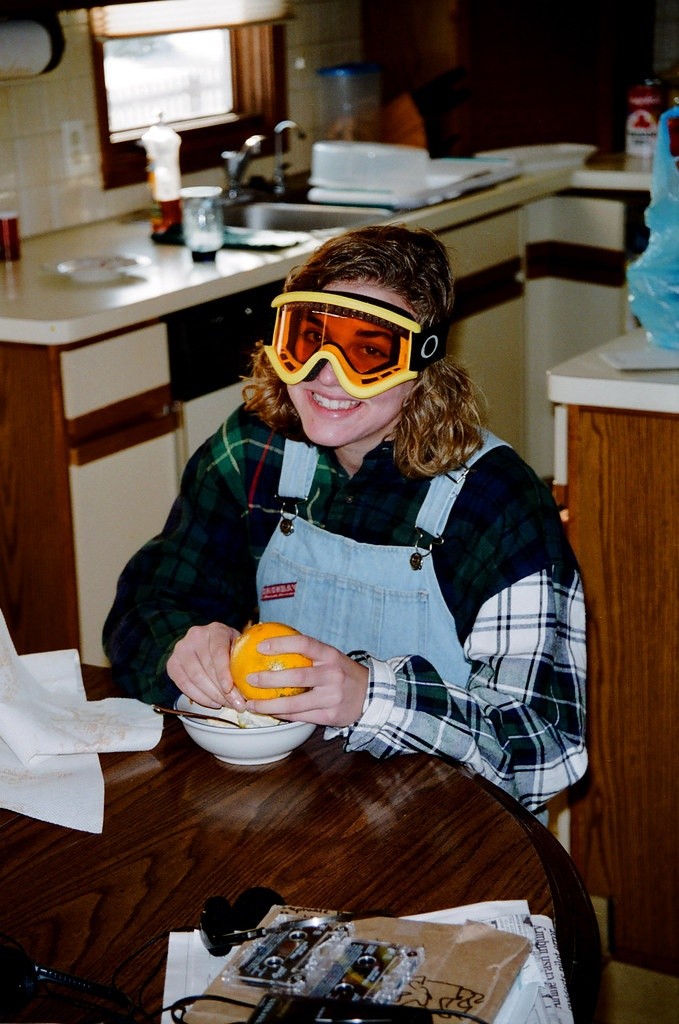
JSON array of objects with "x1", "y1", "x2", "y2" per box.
[
  {"x1": 309, "y1": 141, "x2": 430, "y2": 191},
  {"x1": 173, "y1": 692, "x2": 318, "y2": 765}
]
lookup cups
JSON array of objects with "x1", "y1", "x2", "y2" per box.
[{"x1": 179, "y1": 187, "x2": 225, "y2": 251}]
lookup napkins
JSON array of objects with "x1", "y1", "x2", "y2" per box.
[{"x1": 0, "y1": 608, "x2": 164, "y2": 765}]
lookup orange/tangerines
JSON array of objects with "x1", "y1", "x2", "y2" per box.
[{"x1": 231, "y1": 621, "x2": 315, "y2": 702}]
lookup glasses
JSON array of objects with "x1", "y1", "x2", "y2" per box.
[{"x1": 264, "y1": 291, "x2": 422, "y2": 400}]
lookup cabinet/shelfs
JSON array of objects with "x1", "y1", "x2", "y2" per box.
[
  {"x1": 551, "y1": 406, "x2": 679, "y2": 977},
  {"x1": 0, "y1": 318, "x2": 179, "y2": 668},
  {"x1": 437, "y1": 208, "x2": 527, "y2": 459},
  {"x1": 361, "y1": 0, "x2": 655, "y2": 155},
  {"x1": 520, "y1": 187, "x2": 652, "y2": 479}
]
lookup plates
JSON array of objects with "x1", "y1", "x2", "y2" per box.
[
  {"x1": 475, "y1": 143, "x2": 597, "y2": 173},
  {"x1": 58, "y1": 254, "x2": 147, "y2": 284}
]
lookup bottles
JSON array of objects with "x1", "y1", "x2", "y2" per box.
[{"x1": 139, "y1": 111, "x2": 182, "y2": 203}]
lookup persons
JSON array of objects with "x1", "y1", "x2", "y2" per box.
[{"x1": 101, "y1": 225, "x2": 589, "y2": 827}]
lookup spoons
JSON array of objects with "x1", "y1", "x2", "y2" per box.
[{"x1": 153, "y1": 705, "x2": 280, "y2": 729}]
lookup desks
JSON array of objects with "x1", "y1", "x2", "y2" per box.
[{"x1": 1, "y1": 666, "x2": 607, "y2": 1024}]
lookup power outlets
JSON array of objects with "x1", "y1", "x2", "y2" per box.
[{"x1": 62, "y1": 120, "x2": 90, "y2": 177}]
